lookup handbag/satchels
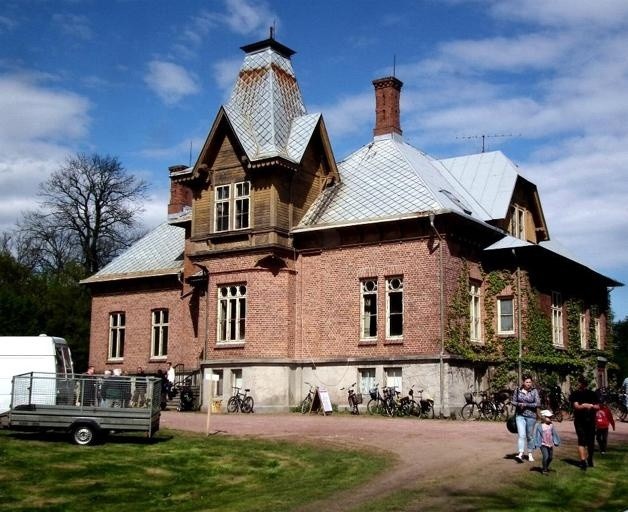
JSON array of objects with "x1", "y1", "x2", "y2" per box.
[{"x1": 507, "y1": 418, "x2": 518, "y2": 433}]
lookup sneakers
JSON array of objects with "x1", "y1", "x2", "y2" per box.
[
  {"x1": 601, "y1": 450, "x2": 606, "y2": 455},
  {"x1": 579, "y1": 456, "x2": 594, "y2": 470},
  {"x1": 543, "y1": 468, "x2": 548, "y2": 474},
  {"x1": 515, "y1": 453, "x2": 535, "y2": 462}
]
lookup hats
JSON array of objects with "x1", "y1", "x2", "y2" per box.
[
  {"x1": 578, "y1": 378, "x2": 587, "y2": 385},
  {"x1": 541, "y1": 410, "x2": 553, "y2": 417}
]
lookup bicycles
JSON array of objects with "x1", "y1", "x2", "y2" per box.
[
  {"x1": 597, "y1": 385, "x2": 628, "y2": 423},
  {"x1": 537, "y1": 383, "x2": 570, "y2": 421},
  {"x1": 460, "y1": 385, "x2": 512, "y2": 420},
  {"x1": 177, "y1": 385, "x2": 194, "y2": 411},
  {"x1": 227, "y1": 387, "x2": 254, "y2": 413},
  {"x1": 340, "y1": 383, "x2": 434, "y2": 419},
  {"x1": 301, "y1": 381, "x2": 333, "y2": 416}
]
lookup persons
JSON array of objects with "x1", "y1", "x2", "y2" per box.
[
  {"x1": 595, "y1": 393, "x2": 615, "y2": 455},
  {"x1": 510, "y1": 374, "x2": 542, "y2": 464},
  {"x1": 571, "y1": 376, "x2": 600, "y2": 471},
  {"x1": 73, "y1": 361, "x2": 176, "y2": 410},
  {"x1": 535, "y1": 409, "x2": 561, "y2": 475}
]
